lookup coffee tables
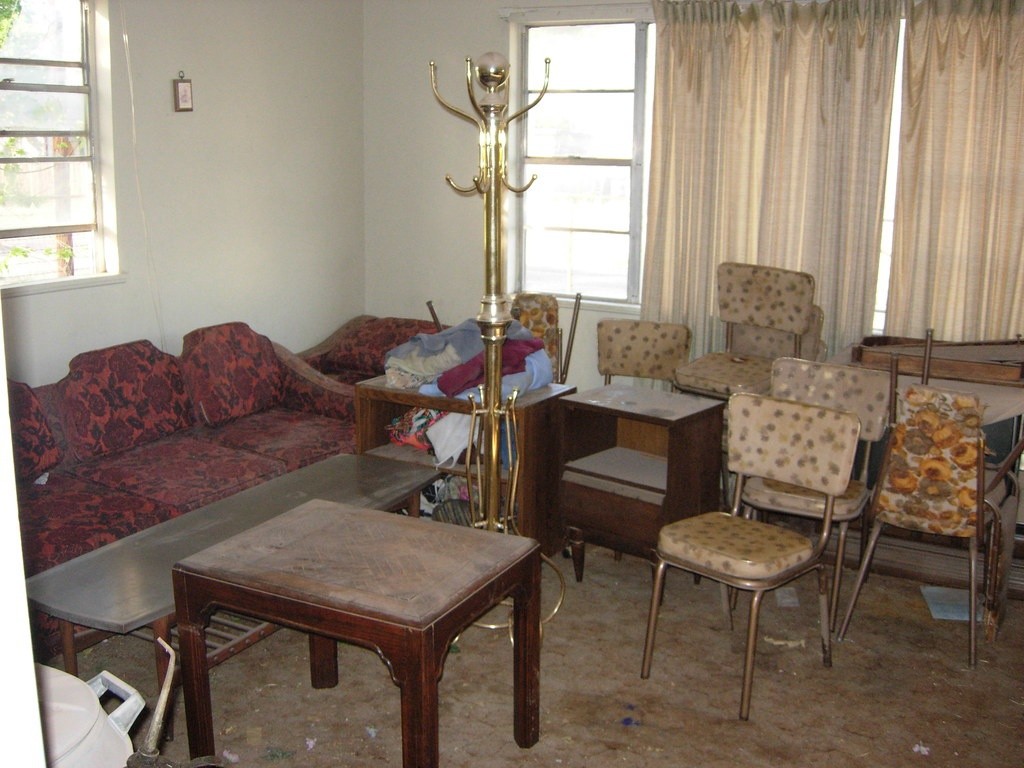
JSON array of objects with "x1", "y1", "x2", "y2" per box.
[{"x1": 32, "y1": 453, "x2": 445, "y2": 740}]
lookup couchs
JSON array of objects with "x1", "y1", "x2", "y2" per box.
[{"x1": 6, "y1": 316, "x2": 452, "y2": 659}]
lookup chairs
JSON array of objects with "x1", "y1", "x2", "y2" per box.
[{"x1": 597, "y1": 262, "x2": 1024, "y2": 723}]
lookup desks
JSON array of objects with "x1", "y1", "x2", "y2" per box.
[{"x1": 171, "y1": 499, "x2": 543, "y2": 768}]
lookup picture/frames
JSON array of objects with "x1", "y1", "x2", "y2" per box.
[{"x1": 173, "y1": 79, "x2": 193, "y2": 112}]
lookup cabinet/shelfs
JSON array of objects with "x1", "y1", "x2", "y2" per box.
[
  {"x1": 354, "y1": 374, "x2": 578, "y2": 560},
  {"x1": 557, "y1": 383, "x2": 726, "y2": 604}
]
